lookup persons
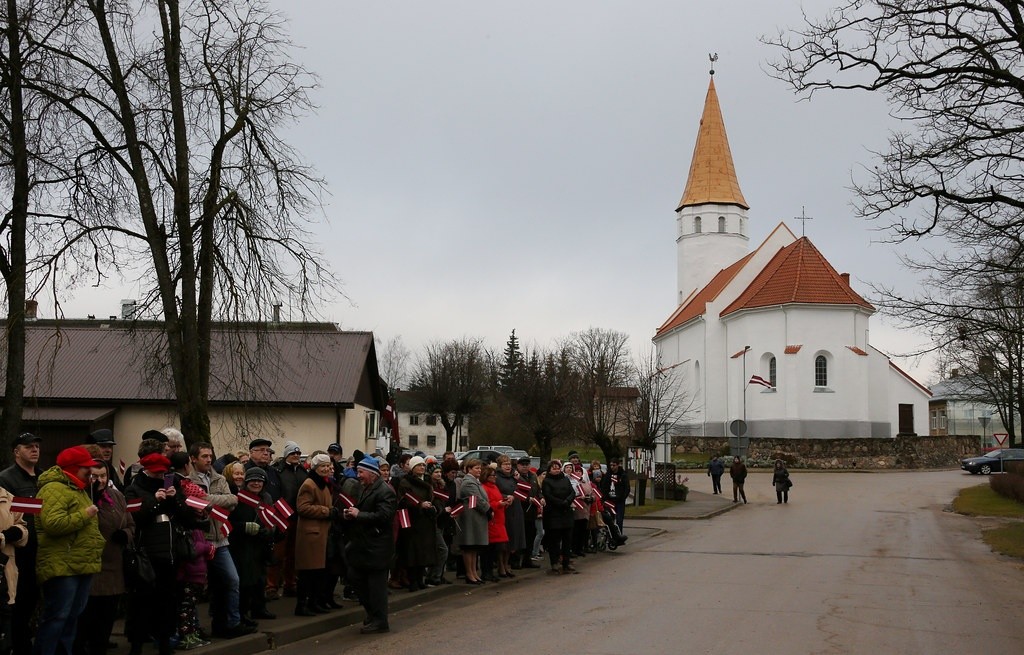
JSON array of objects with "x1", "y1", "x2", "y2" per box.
[
  {"x1": 707, "y1": 454, "x2": 724, "y2": 494},
  {"x1": 587, "y1": 457, "x2": 630, "y2": 545},
  {"x1": 772, "y1": 459, "x2": 789, "y2": 504},
  {"x1": 0, "y1": 428, "x2": 592, "y2": 655},
  {"x1": 730, "y1": 455, "x2": 747, "y2": 504}
]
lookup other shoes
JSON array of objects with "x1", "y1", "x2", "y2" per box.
[{"x1": 108, "y1": 534, "x2": 630, "y2": 655}]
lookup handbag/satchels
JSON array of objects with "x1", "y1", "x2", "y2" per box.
[
  {"x1": 123, "y1": 526, "x2": 156, "y2": 582},
  {"x1": 587, "y1": 497, "x2": 605, "y2": 529}
]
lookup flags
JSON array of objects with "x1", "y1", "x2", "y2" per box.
[
  {"x1": 384, "y1": 393, "x2": 396, "y2": 423},
  {"x1": 399, "y1": 508, "x2": 411, "y2": 528},
  {"x1": 90, "y1": 471, "x2": 92, "y2": 483},
  {"x1": 238, "y1": 488, "x2": 260, "y2": 509},
  {"x1": 338, "y1": 490, "x2": 357, "y2": 509},
  {"x1": 125, "y1": 497, "x2": 142, "y2": 513},
  {"x1": 185, "y1": 494, "x2": 211, "y2": 512},
  {"x1": 572, "y1": 471, "x2": 616, "y2": 511},
  {"x1": 749, "y1": 375, "x2": 772, "y2": 389},
  {"x1": 210, "y1": 504, "x2": 230, "y2": 523},
  {"x1": 120, "y1": 460, "x2": 125, "y2": 475},
  {"x1": 274, "y1": 497, "x2": 294, "y2": 518},
  {"x1": 257, "y1": 507, "x2": 275, "y2": 529},
  {"x1": 258, "y1": 500, "x2": 271, "y2": 509},
  {"x1": 390, "y1": 413, "x2": 400, "y2": 446},
  {"x1": 433, "y1": 490, "x2": 477, "y2": 515},
  {"x1": 220, "y1": 521, "x2": 233, "y2": 538},
  {"x1": 513, "y1": 480, "x2": 541, "y2": 508},
  {"x1": 404, "y1": 490, "x2": 421, "y2": 506},
  {"x1": 10, "y1": 496, "x2": 43, "y2": 515},
  {"x1": 610, "y1": 476, "x2": 618, "y2": 483},
  {"x1": 265, "y1": 505, "x2": 289, "y2": 532}
]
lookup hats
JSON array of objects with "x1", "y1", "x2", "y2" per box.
[
  {"x1": 140, "y1": 453, "x2": 171, "y2": 472},
  {"x1": 138, "y1": 438, "x2": 163, "y2": 458},
  {"x1": 88, "y1": 429, "x2": 117, "y2": 444},
  {"x1": 245, "y1": 439, "x2": 602, "y2": 481},
  {"x1": 180, "y1": 480, "x2": 209, "y2": 498},
  {"x1": 142, "y1": 430, "x2": 169, "y2": 441},
  {"x1": 56, "y1": 446, "x2": 99, "y2": 467},
  {"x1": 11, "y1": 432, "x2": 43, "y2": 448}
]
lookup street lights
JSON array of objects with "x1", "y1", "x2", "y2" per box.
[{"x1": 743, "y1": 345, "x2": 751, "y2": 468}]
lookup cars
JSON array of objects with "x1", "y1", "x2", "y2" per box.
[
  {"x1": 960, "y1": 448, "x2": 1024, "y2": 475},
  {"x1": 456, "y1": 445, "x2": 540, "y2": 470}
]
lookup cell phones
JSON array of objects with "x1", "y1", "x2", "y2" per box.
[{"x1": 164, "y1": 474, "x2": 175, "y2": 490}]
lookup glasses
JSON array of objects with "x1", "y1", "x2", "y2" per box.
[{"x1": 92, "y1": 474, "x2": 107, "y2": 480}]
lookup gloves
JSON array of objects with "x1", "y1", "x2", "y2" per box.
[
  {"x1": 2, "y1": 526, "x2": 23, "y2": 543},
  {"x1": 246, "y1": 521, "x2": 260, "y2": 534},
  {"x1": 207, "y1": 541, "x2": 216, "y2": 560},
  {"x1": 328, "y1": 506, "x2": 339, "y2": 519},
  {"x1": 540, "y1": 498, "x2": 546, "y2": 506},
  {"x1": 88, "y1": 480, "x2": 104, "y2": 503},
  {"x1": 529, "y1": 497, "x2": 534, "y2": 504},
  {"x1": 112, "y1": 528, "x2": 128, "y2": 546}
]
